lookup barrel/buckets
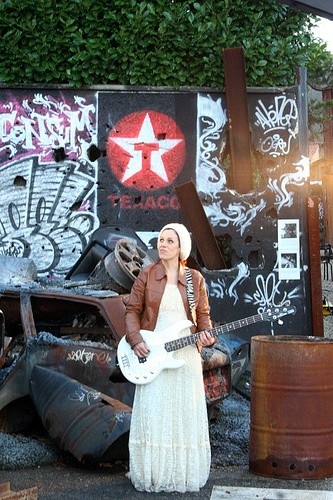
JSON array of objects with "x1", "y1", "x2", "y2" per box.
[{"x1": 249, "y1": 334, "x2": 331, "y2": 481}]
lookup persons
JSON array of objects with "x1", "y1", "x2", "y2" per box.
[{"x1": 124, "y1": 223, "x2": 217, "y2": 492}]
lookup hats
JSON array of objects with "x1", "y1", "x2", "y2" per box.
[{"x1": 157, "y1": 222, "x2": 192, "y2": 262}]
